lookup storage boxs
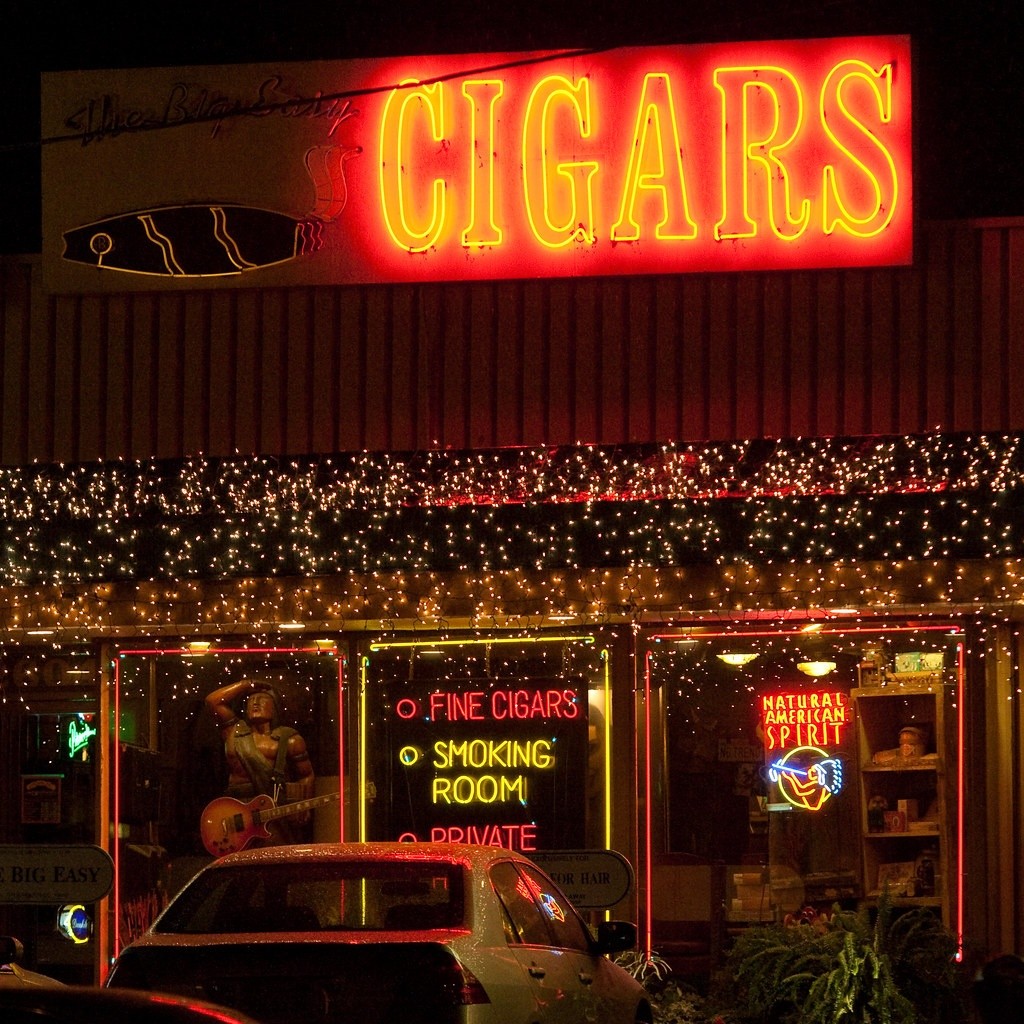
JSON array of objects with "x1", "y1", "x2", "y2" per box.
[{"x1": 732, "y1": 874, "x2": 771, "y2": 910}]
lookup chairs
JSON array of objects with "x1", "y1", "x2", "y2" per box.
[
  {"x1": 225, "y1": 906, "x2": 322, "y2": 932},
  {"x1": 636, "y1": 855, "x2": 722, "y2": 999},
  {"x1": 382, "y1": 902, "x2": 455, "y2": 929}
]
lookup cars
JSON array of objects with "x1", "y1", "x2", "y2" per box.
[
  {"x1": 0, "y1": 931, "x2": 259, "y2": 1024},
  {"x1": 102, "y1": 840, "x2": 663, "y2": 1023}
]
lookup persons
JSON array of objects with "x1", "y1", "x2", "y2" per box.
[
  {"x1": 669, "y1": 743, "x2": 748, "y2": 864},
  {"x1": 204, "y1": 678, "x2": 313, "y2": 906}
]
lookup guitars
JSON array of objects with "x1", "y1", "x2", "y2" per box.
[{"x1": 200, "y1": 781, "x2": 378, "y2": 857}]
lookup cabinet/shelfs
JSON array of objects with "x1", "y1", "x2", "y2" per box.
[{"x1": 851, "y1": 685, "x2": 973, "y2": 954}]
